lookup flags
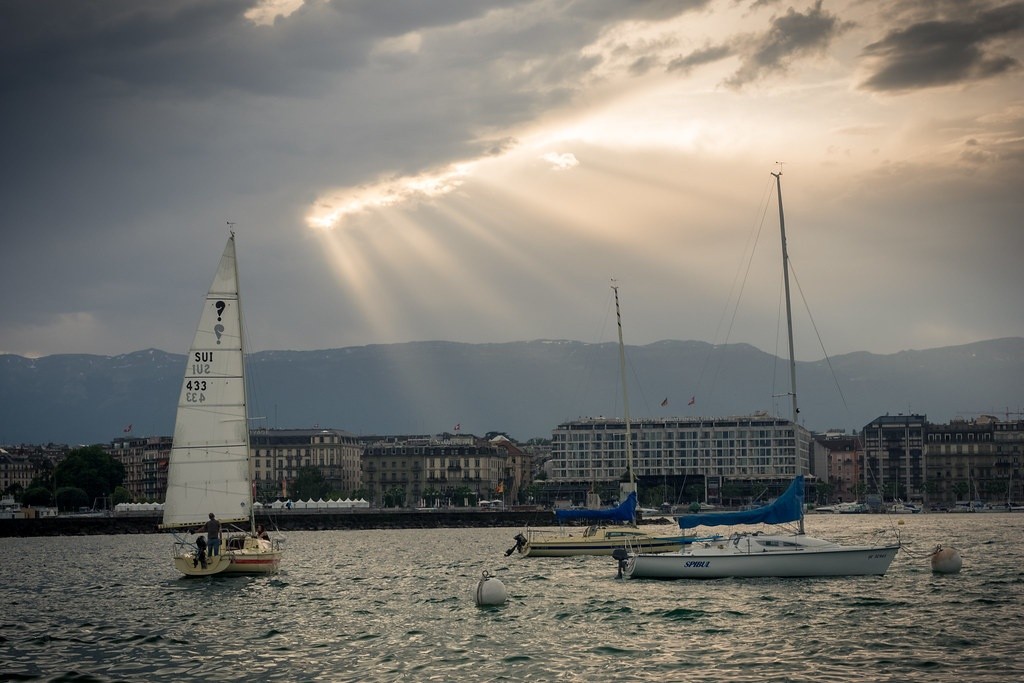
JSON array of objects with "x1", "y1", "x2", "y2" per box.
[
  {"x1": 661, "y1": 398, "x2": 669, "y2": 407},
  {"x1": 495, "y1": 481, "x2": 503, "y2": 493},
  {"x1": 688, "y1": 396, "x2": 694, "y2": 405}
]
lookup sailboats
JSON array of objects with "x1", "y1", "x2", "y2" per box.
[
  {"x1": 611, "y1": 168, "x2": 905, "y2": 583},
  {"x1": 504, "y1": 277, "x2": 699, "y2": 557},
  {"x1": 155, "y1": 231, "x2": 283, "y2": 578}
]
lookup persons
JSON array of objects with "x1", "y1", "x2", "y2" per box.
[
  {"x1": 288, "y1": 500, "x2": 291, "y2": 510},
  {"x1": 257, "y1": 524, "x2": 270, "y2": 541},
  {"x1": 190, "y1": 512, "x2": 223, "y2": 556}
]
